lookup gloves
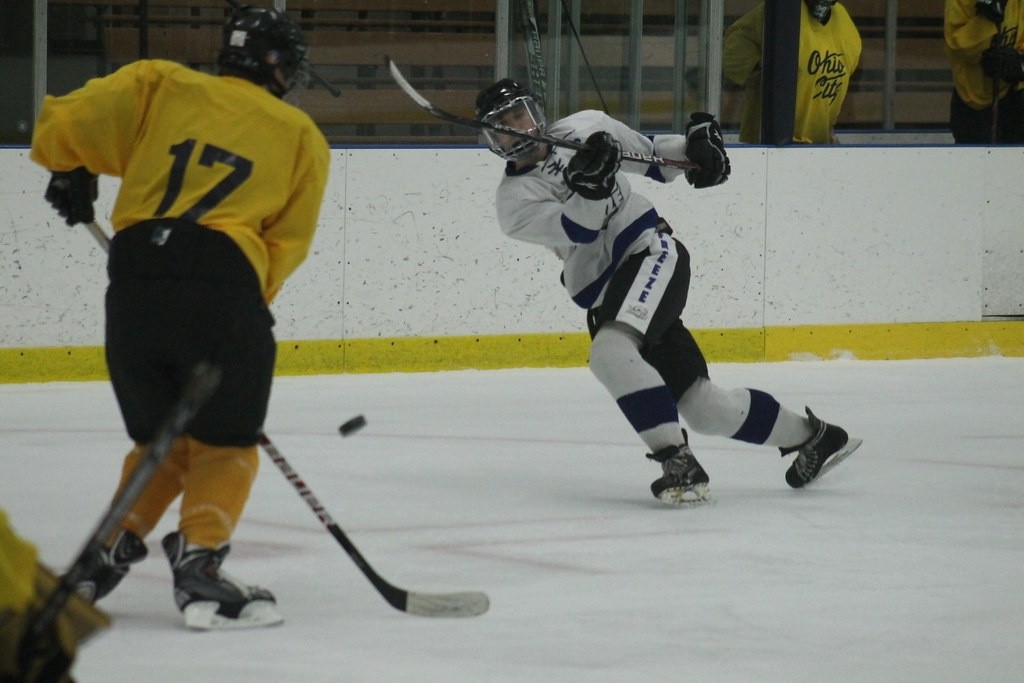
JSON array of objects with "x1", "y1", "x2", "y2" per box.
[
  {"x1": 563, "y1": 131, "x2": 622, "y2": 200},
  {"x1": 685, "y1": 112, "x2": 731, "y2": 189},
  {"x1": 17, "y1": 602, "x2": 77, "y2": 683},
  {"x1": 980, "y1": 47, "x2": 1024, "y2": 82},
  {"x1": 976, "y1": 0, "x2": 1007, "y2": 22},
  {"x1": 45, "y1": 167, "x2": 98, "y2": 226}
]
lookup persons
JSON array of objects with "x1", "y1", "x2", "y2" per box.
[
  {"x1": 28, "y1": 5, "x2": 330, "y2": 634},
  {"x1": 719, "y1": 0, "x2": 864, "y2": 144},
  {"x1": 944, "y1": 0, "x2": 1024, "y2": 145},
  {"x1": 472, "y1": 77, "x2": 865, "y2": 508},
  {"x1": 0, "y1": 518, "x2": 112, "y2": 683}
]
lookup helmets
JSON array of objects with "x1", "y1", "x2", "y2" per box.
[
  {"x1": 213, "y1": 5, "x2": 307, "y2": 98},
  {"x1": 475, "y1": 78, "x2": 547, "y2": 161}
]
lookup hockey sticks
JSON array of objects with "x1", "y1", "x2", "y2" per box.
[
  {"x1": 381, "y1": 52, "x2": 703, "y2": 171},
  {"x1": 1, "y1": 299, "x2": 276, "y2": 683},
  {"x1": 85, "y1": 222, "x2": 491, "y2": 618}
]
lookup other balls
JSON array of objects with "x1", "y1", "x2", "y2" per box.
[{"x1": 338, "y1": 416, "x2": 365, "y2": 436}]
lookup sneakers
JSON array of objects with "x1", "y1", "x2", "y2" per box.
[
  {"x1": 163, "y1": 531, "x2": 284, "y2": 629},
  {"x1": 64, "y1": 528, "x2": 148, "y2": 607},
  {"x1": 647, "y1": 428, "x2": 714, "y2": 508},
  {"x1": 779, "y1": 406, "x2": 863, "y2": 489}
]
